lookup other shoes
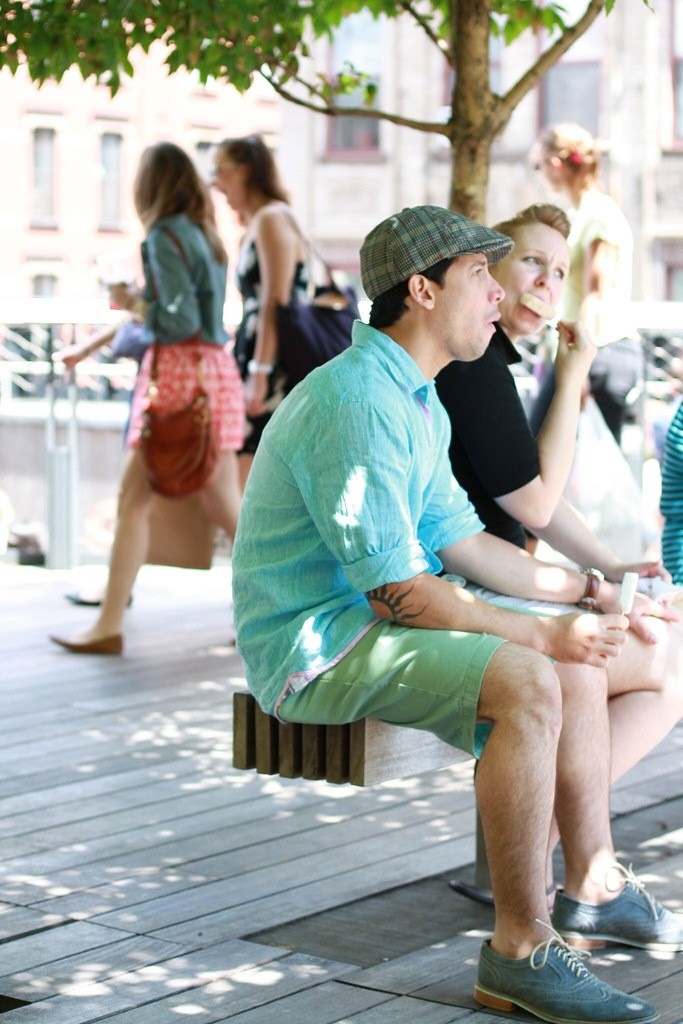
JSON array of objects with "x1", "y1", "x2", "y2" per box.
[
  {"x1": 64, "y1": 592, "x2": 134, "y2": 608},
  {"x1": 48, "y1": 630, "x2": 124, "y2": 654}
]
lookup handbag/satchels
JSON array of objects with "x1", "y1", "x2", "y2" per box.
[
  {"x1": 274, "y1": 283, "x2": 360, "y2": 382},
  {"x1": 139, "y1": 393, "x2": 220, "y2": 501}
]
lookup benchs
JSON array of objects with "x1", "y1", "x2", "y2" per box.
[{"x1": 233, "y1": 687, "x2": 496, "y2": 905}]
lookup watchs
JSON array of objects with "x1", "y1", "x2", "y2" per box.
[{"x1": 578, "y1": 567, "x2": 604, "y2": 610}]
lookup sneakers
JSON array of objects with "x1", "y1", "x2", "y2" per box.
[
  {"x1": 473, "y1": 937, "x2": 659, "y2": 1023},
  {"x1": 553, "y1": 881, "x2": 683, "y2": 951}
]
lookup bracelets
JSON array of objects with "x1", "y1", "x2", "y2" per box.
[
  {"x1": 247, "y1": 359, "x2": 273, "y2": 376},
  {"x1": 134, "y1": 300, "x2": 147, "y2": 322}
]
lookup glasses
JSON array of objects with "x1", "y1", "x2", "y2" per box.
[
  {"x1": 531, "y1": 153, "x2": 564, "y2": 170},
  {"x1": 211, "y1": 159, "x2": 241, "y2": 177}
]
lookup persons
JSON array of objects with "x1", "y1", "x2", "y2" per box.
[
  {"x1": 47, "y1": 131, "x2": 315, "y2": 653},
  {"x1": 230, "y1": 123, "x2": 683, "y2": 1024}
]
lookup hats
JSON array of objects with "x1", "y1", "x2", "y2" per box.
[{"x1": 360, "y1": 205, "x2": 515, "y2": 301}]
